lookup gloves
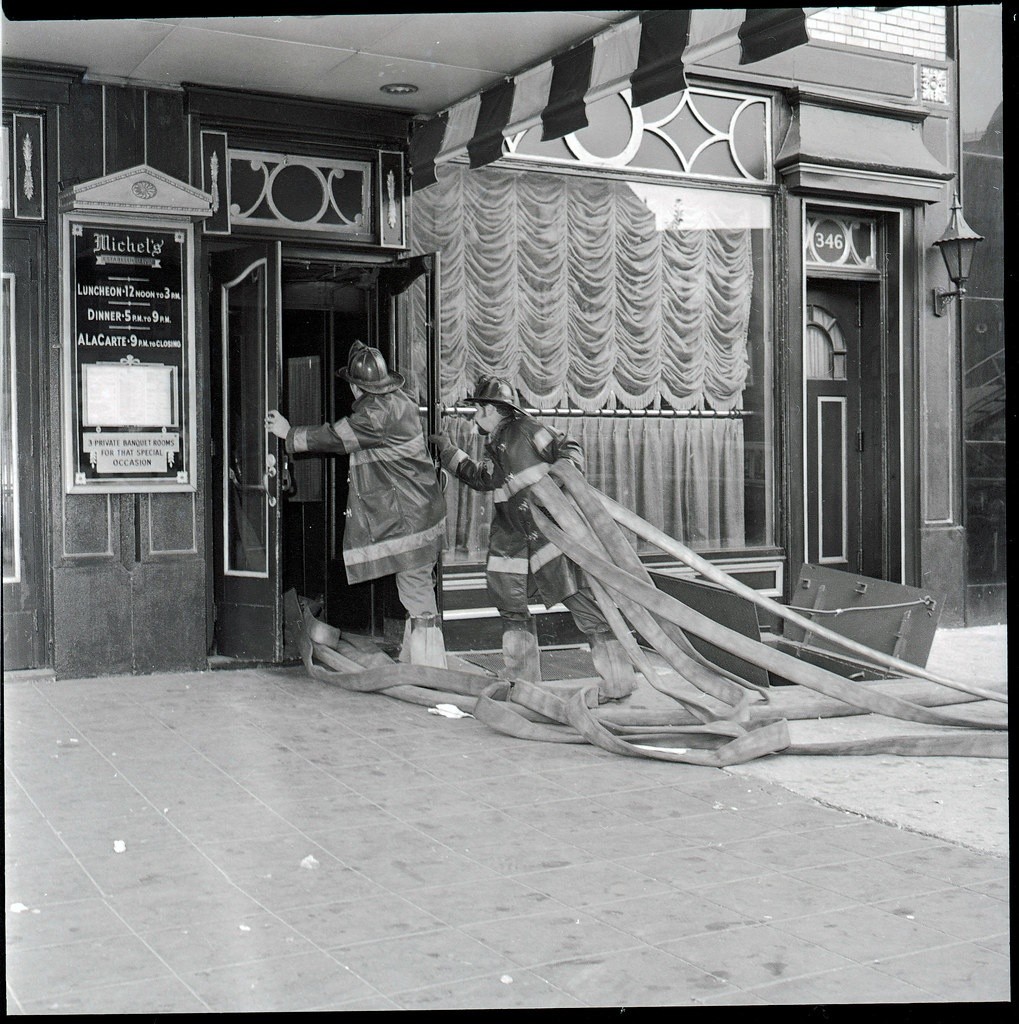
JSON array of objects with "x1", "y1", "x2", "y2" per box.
[{"x1": 428, "y1": 430, "x2": 458, "y2": 469}]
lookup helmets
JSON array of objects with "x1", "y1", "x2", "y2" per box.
[
  {"x1": 334, "y1": 341, "x2": 405, "y2": 393},
  {"x1": 463, "y1": 376, "x2": 533, "y2": 418}
]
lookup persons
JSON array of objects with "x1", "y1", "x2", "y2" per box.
[
  {"x1": 428, "y1": 377, "x2": 637, "y2": 705},
  {"x1": 264, "y1": 346, "x2": 448, "y2": 671}
]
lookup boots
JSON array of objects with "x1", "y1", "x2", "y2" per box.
[
  {"x1": 493, "y1": 615, "x2": 541, "y2": 681},
  {"x1": 586, "y1": 632, "x2": 636, "y2": 704},
  {"x1": 408, "y1": 613, "x2": 447, "y2": 668}
]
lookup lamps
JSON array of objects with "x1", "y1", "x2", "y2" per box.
[{"x1": 931, "y1": 189, "x2": 985, "y2": 317}]
